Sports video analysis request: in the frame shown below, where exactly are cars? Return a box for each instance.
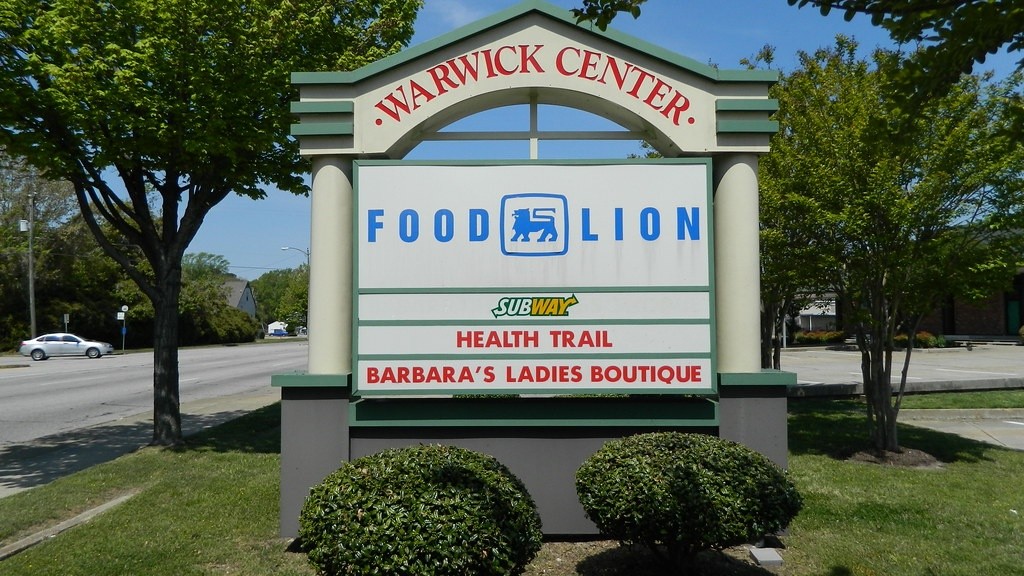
[19,333,114,361]
[270,329,288,337]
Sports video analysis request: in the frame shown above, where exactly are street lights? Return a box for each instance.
[281,246,310,286]
